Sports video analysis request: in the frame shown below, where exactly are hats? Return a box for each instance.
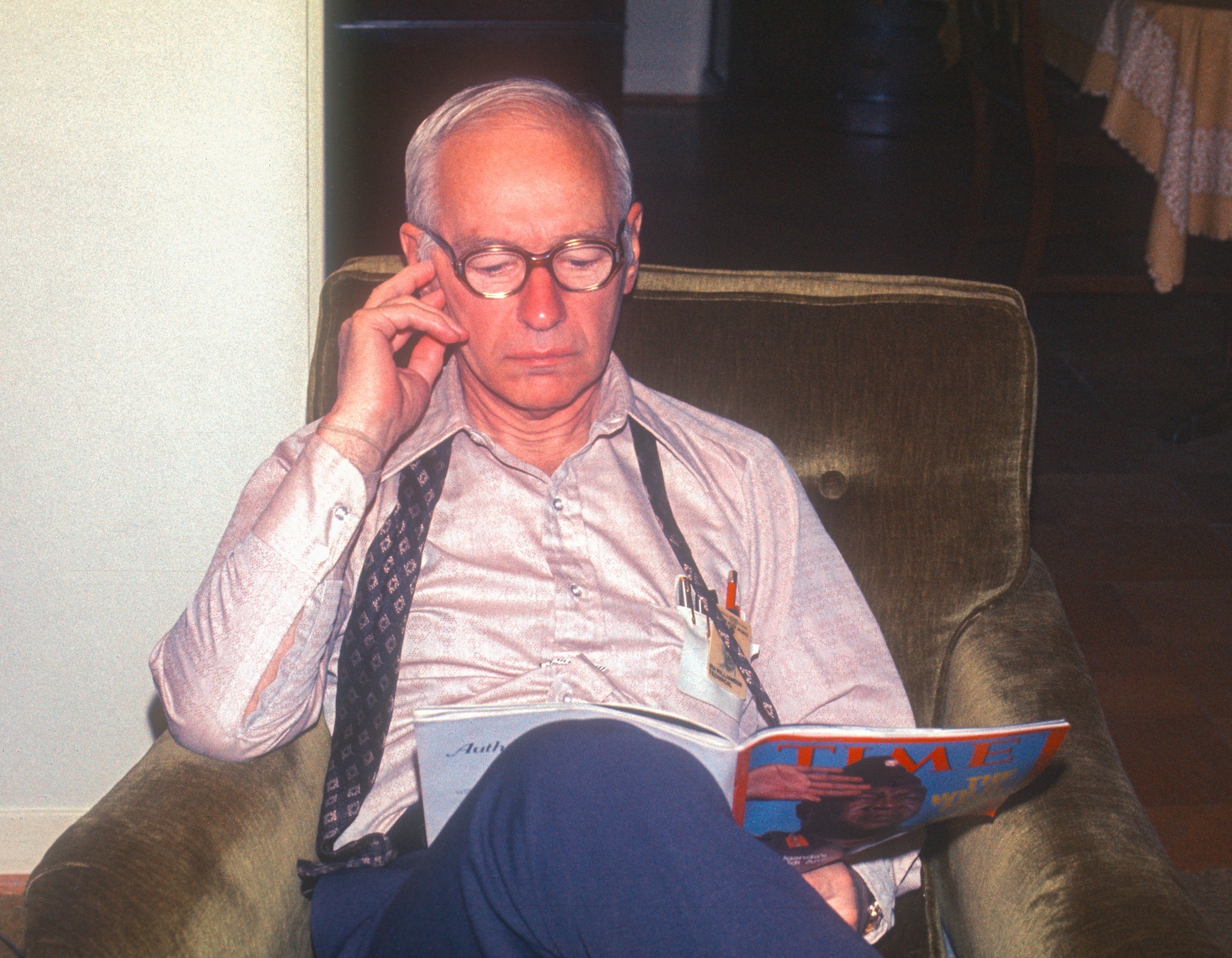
[837,754,921,786]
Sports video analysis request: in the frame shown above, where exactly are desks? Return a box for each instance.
[935,0,1232,294]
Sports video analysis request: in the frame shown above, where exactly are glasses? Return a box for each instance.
[417,221,628,302]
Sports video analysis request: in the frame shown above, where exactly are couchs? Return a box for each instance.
[14,253,1213,958]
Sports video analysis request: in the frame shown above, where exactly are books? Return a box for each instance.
[412,703,1070,874]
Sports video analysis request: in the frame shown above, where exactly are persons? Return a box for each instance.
[148,78,922,957]
[747,757,927,856]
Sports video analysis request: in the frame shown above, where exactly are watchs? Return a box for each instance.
[851,867,882,934]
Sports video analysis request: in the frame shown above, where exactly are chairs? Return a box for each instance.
[960,0,1144,311]
